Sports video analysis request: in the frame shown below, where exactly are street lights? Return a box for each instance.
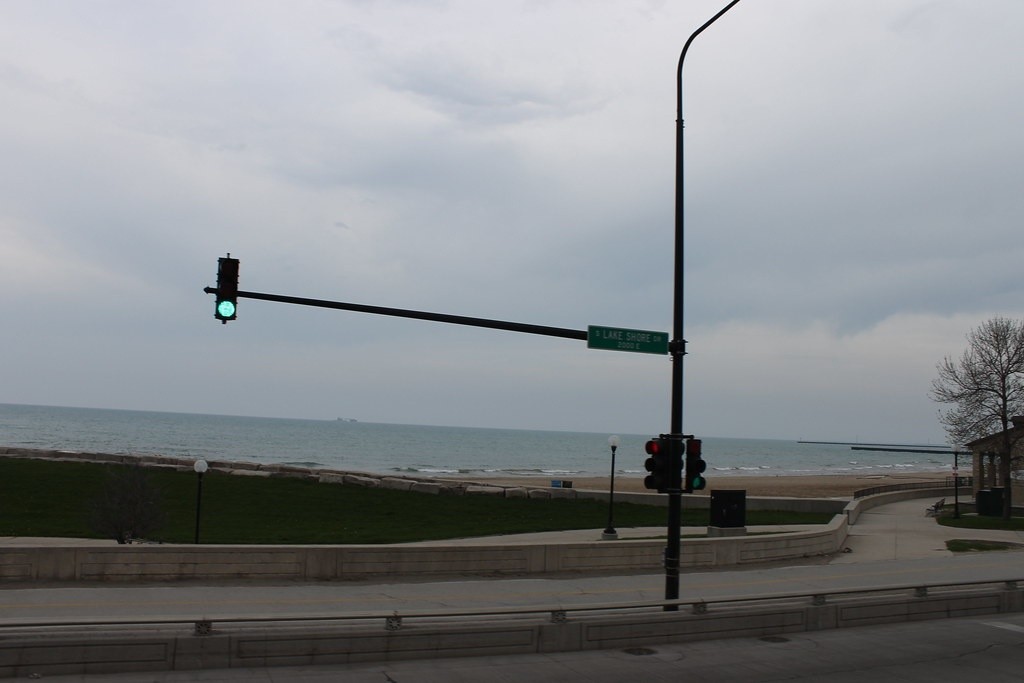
[603,434,619,534]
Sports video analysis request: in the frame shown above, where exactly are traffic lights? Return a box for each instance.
[684,437,708,494]
[644,437,670,495]
[214,256,240,322]
